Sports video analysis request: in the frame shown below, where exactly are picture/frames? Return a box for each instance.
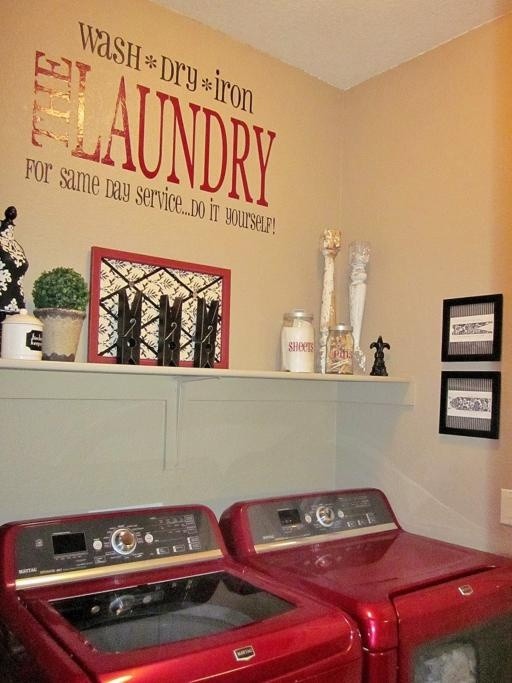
[438,370,501,440]
[440,292,504,361]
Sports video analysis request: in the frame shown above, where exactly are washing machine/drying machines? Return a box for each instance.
[216,486,512,683]
[0,505,364,683]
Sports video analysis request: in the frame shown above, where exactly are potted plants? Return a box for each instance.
[32,266,91,362]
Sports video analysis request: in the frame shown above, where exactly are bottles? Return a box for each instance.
[279,308,317,376]
[323,320,354,377]
[0,306,45,362]
